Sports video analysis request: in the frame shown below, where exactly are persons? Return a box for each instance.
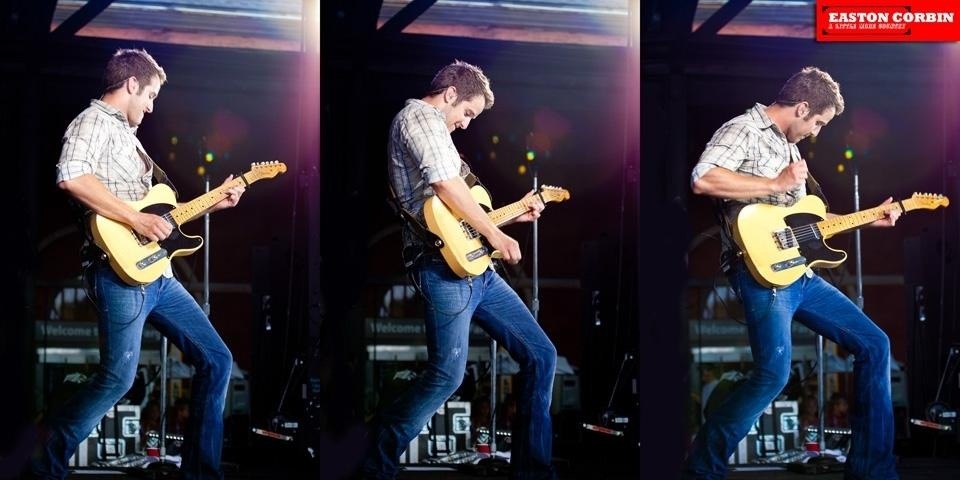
[366,60,561,479]
[680,67,906,480]
[32,46,248,480]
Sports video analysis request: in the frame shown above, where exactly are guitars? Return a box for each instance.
[732,191,950,290]
[424,184,570,278]
[90,160,287,286]
